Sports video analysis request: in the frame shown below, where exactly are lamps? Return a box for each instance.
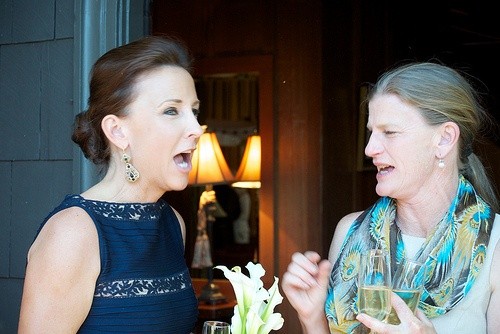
[232,131,261,269]
[186,131,236,305]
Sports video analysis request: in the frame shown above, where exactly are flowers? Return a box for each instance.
[215,264,286,332]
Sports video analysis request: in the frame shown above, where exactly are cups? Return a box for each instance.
[389,258,425,325]
[203,321,230,334]
[356,249,390,334]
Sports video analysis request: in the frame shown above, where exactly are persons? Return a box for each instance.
[18,36,203,334]
[282,62,500,334]
[167,185,258,278]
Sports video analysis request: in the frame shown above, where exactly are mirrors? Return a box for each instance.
[179,64,275,283]
